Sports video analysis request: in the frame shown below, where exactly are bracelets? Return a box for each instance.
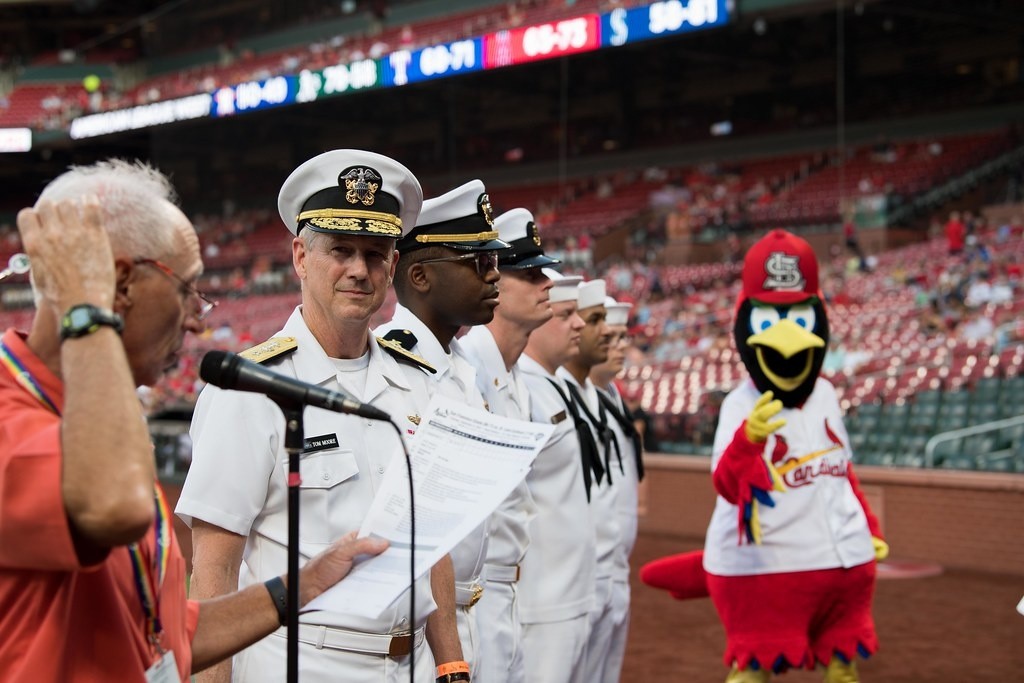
[435,661,470,678]
[436,672,470,683]
[265,577,289,627]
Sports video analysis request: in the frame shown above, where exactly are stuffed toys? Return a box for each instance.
[639,229,891,683]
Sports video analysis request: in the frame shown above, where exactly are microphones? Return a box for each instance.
[199,350,392,423]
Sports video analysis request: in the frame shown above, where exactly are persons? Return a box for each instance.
[607,264,743,360]
[648,161,773,238]
[0,148,645,683]
[910,209,1024,353]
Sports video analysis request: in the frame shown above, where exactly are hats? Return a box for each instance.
[603,296,633,326]
[278,149,424,244]
[575,279,607,310]
[541,267,583,303]
[489,207,562,272]
[397,180,513,251]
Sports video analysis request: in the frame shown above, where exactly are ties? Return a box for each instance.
[549,378,603,504]
[567,381,625,487]
[594,386,645,483]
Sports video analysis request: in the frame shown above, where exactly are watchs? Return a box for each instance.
[59,304,126,340]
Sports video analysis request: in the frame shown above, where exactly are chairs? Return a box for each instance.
[623,232,1024,472]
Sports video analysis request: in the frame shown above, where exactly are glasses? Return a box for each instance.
[129,257,219,321]
[415,249,498,279]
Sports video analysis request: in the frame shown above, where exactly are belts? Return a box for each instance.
[271,622,425,663]
[477,564,521,584]
[454,579,484,606]
